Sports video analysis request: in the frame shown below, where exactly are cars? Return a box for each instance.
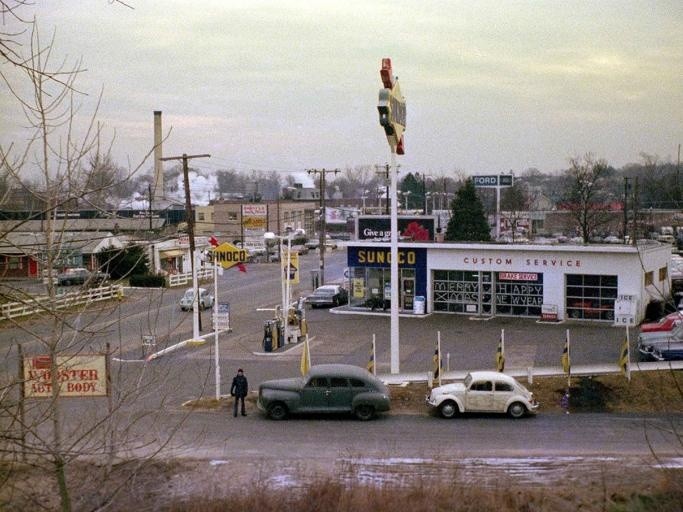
[304,284,348,309]
[424,371,541,419]
[490,218,683,245]
[41,267,111,287]
[236,238,341,263]
[255,363,391,421]
[636,309,683,361]
[179,287,215,312]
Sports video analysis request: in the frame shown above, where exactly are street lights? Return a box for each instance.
[263,228,306,346]
[361,190,447,216]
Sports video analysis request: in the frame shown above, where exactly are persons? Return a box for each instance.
[229,367,249,417]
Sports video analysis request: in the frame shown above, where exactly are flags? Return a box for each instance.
[560,336,571,377]
[429,331,447,385]
[494,331,506,371]
[617,324,632,379]
[299,337,311,375]
[367,340,375,374]
